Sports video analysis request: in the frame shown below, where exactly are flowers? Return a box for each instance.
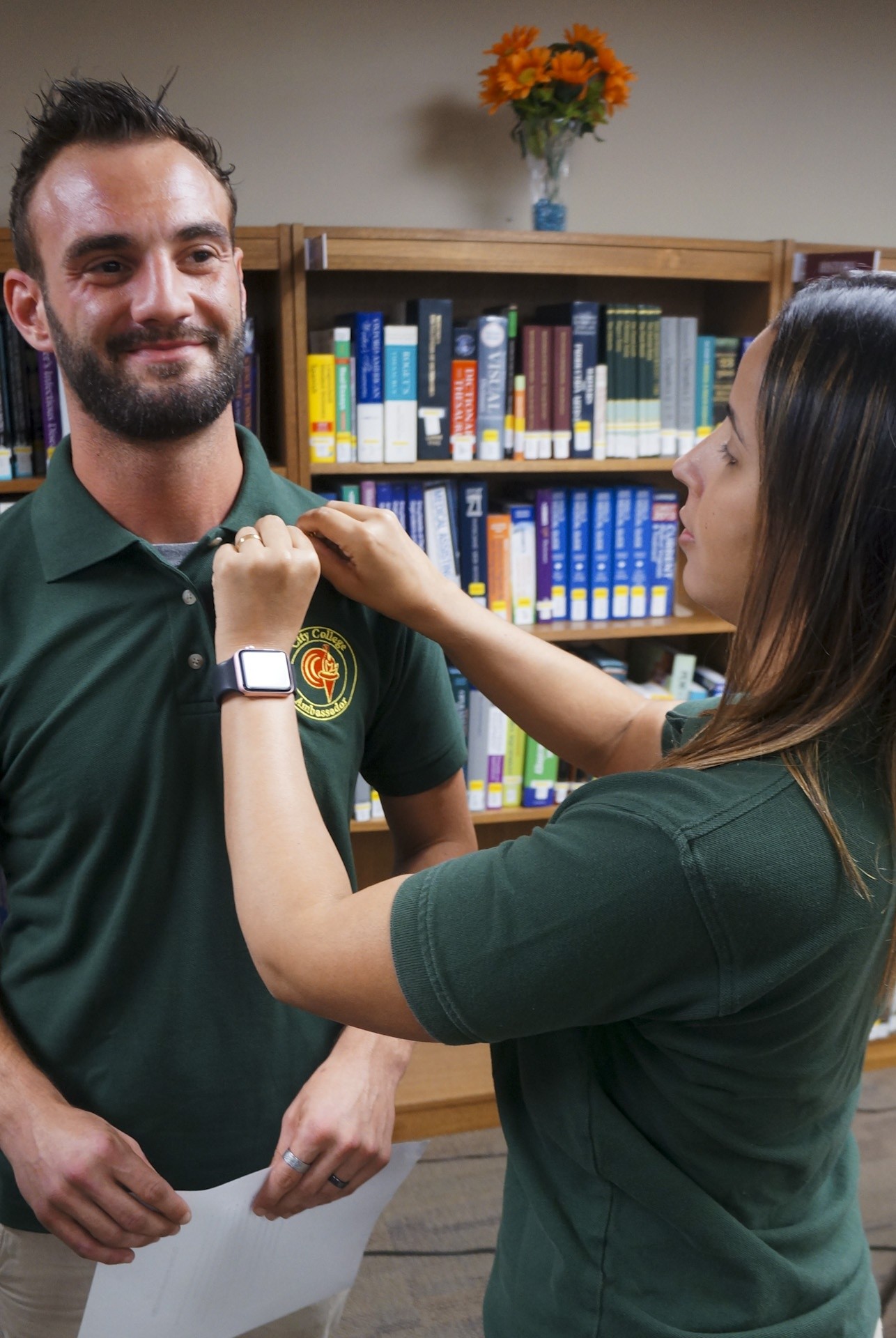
[476,21,640,204]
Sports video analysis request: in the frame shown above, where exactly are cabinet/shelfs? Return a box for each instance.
[0,223,896,1144]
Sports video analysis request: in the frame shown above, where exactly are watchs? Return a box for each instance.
[213,645,296,706]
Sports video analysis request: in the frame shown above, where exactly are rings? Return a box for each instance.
[235,534,262,552]
[282,1148,311,1175]
[328,1174,348,1189]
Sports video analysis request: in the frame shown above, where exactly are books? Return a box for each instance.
[303,473,696,624]
[0,272,282,483]
[306,274,766,465]
[346,644,731,824]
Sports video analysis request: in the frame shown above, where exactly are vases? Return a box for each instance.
[519,119,581,231]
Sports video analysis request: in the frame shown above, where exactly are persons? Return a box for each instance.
[0,75,479,1338]
[211,266,896,1338]
